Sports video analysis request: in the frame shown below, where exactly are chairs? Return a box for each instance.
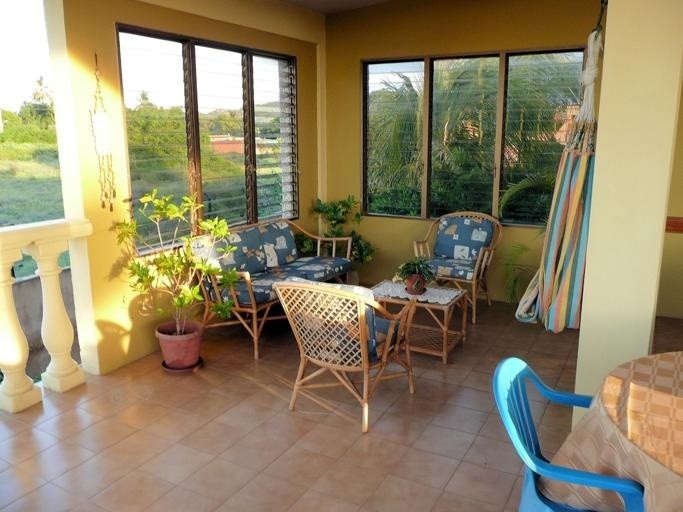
[493,357,645,512]
[413,211,503,324]
[272,276,417,432]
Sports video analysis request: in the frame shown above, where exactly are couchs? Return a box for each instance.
[183,218,353,360]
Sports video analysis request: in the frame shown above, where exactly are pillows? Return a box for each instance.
[433,216,494,262]
[283,276,376,356]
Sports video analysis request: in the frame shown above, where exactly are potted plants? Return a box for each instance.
[392,255,434,294]
[112,189,239,375]
